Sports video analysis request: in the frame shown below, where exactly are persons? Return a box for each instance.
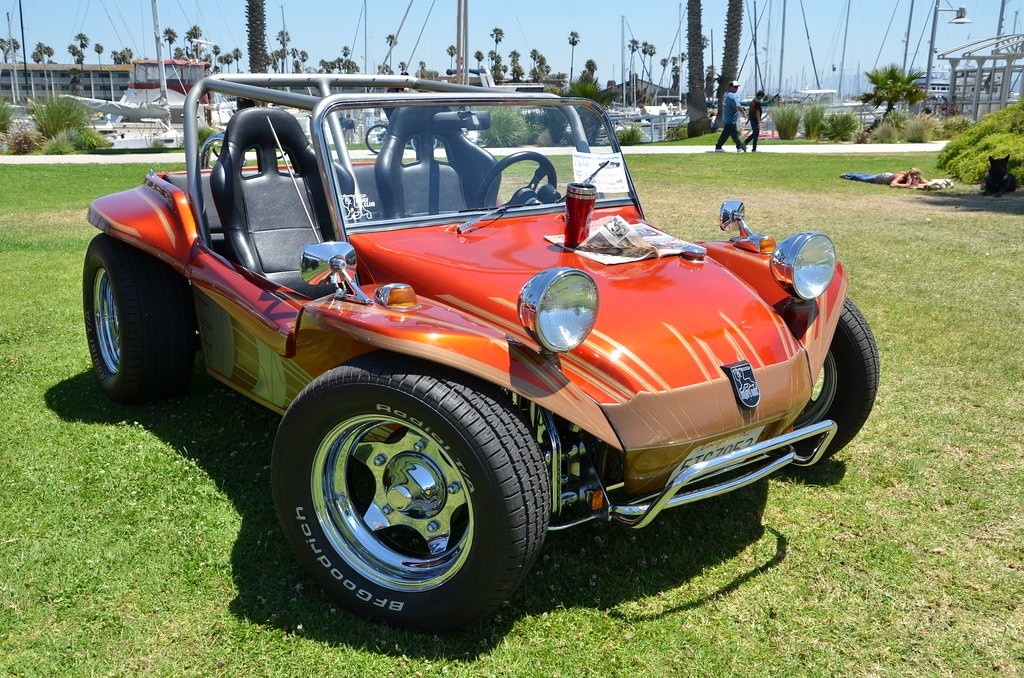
[714,80,748,152]
[839,167,928,188]
[742,90,765,152]
[338,112,355,144]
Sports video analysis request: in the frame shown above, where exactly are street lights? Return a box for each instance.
[924,6,973,108]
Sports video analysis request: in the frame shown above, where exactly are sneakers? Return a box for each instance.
[715,148,725,152]
[737,148,744,154]
[743,144,748,152]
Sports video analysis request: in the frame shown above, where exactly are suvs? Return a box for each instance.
[83,72,881,635]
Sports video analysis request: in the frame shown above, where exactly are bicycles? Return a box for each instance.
[365,124,437,155]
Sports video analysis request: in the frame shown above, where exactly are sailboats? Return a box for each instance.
[1,0,1024,149]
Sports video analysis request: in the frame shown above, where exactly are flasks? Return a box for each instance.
[565,183,596,248]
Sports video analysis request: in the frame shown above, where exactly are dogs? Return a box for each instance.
[981,153,1016,197]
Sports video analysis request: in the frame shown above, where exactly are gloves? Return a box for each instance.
[742,113,746,120]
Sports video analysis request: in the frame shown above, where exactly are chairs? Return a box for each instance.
[372,106,503,220]
[209,107,355,300]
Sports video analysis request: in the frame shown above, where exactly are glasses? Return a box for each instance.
[731,86,738,88]
[912,175,921,180]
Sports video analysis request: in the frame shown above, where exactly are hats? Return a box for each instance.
[758,90,765,94]
[729,81,740,87]
